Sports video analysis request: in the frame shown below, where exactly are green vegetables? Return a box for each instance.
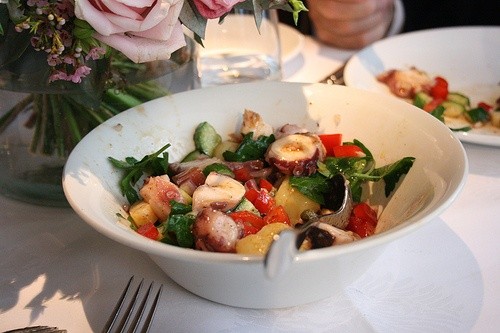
[107,132,416,250]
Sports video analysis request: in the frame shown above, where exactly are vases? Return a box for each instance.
[0,56,192,207]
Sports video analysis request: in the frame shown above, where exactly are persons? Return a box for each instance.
[274,0,500,49]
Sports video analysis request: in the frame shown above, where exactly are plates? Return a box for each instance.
[181,14,305,77]
[343,25,500,149]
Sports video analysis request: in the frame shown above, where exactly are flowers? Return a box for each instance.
[0,0,310,97]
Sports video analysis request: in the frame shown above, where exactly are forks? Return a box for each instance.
[102,274,165,333]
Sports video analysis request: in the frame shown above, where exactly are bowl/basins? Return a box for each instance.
[58,82,468,309]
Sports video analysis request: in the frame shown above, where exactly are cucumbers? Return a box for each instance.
[415,90,490,133]
[193,121,222,157]
